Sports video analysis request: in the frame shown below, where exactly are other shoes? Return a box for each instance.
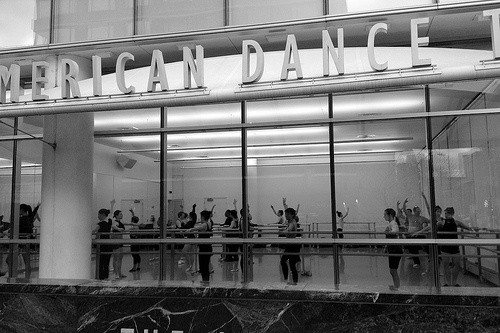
[442,283,461,286]
[248,260,254,265]
[230,268,239,272]
[115,272,127,279]
[2,272,9,278]
[218,257,239,262]
[129,267,140,272]
[413,264,418,267]
[178,259,184,265]
[186,265,192,272]
[210,270,214,274]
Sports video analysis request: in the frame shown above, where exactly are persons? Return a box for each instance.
[0,196,479,291]
